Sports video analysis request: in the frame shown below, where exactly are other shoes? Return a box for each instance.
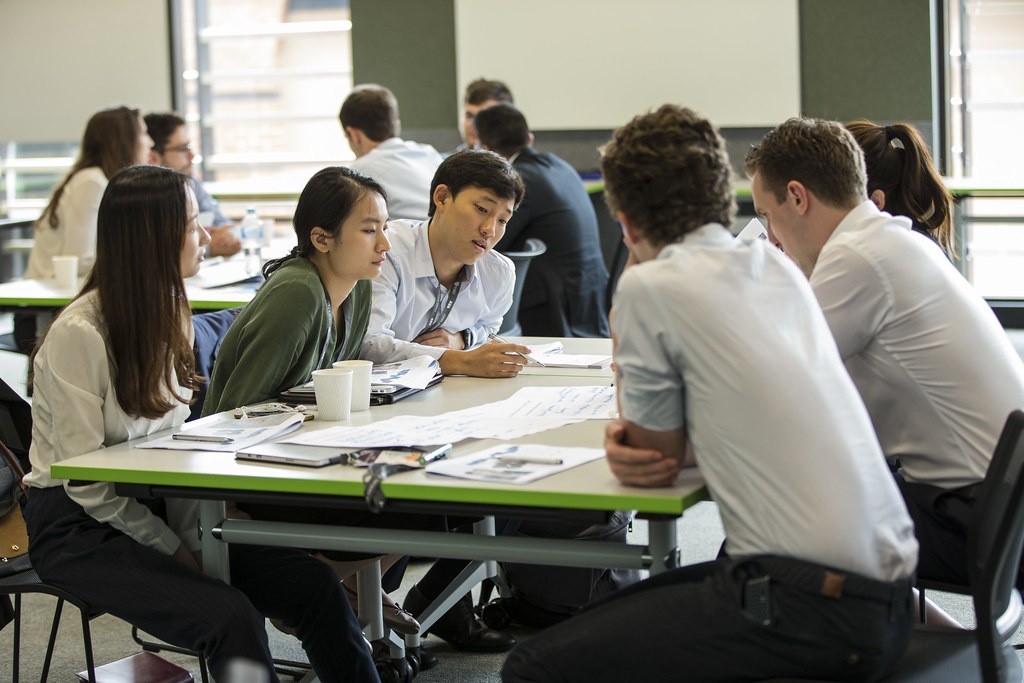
[341,587,420,633]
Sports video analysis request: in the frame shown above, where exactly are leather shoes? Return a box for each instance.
[400,585,517,651]
[395,629,439,670]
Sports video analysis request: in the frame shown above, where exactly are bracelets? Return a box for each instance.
[459,328,472,351]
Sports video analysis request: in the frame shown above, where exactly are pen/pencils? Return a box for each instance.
[417,444,453,465]
[489,333,545,366]
[496,455,563,463]
[172,435,235,442]
[544,362,603,370]
[611,374,617,387]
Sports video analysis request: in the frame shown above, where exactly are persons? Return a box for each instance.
[336,154,518,651]
[465,102,614,342]
[741,113,1023,643]
[138,113,243,260]
[494,99,918,683]
[438,81,524,182]
[184,155,424,653]
[328,85,446,226]
[20,162,392,681]
[10,106,218,361]
[839,120,950,261]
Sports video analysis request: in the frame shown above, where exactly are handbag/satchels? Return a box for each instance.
[0,437,36,576]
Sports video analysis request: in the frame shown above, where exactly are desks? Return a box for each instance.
[0,239,303,334]
[732,177,1024,283]
[50,337,709,683]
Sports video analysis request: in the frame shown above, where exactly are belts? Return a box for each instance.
[764,556,917,604]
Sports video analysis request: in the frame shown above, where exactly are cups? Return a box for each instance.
[311,369,354,422]
[332,360,373,412]
[53,256,80,295]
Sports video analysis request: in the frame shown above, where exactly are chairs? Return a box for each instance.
[497,238,547,337]
[880,410,1024,683]
[0,379,207,683]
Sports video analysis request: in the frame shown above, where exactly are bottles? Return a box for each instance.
[240,209,264,275]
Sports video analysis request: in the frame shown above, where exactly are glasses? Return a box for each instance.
[159,141,194,154]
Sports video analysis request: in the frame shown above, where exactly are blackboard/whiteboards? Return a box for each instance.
[454,0,803,131]
